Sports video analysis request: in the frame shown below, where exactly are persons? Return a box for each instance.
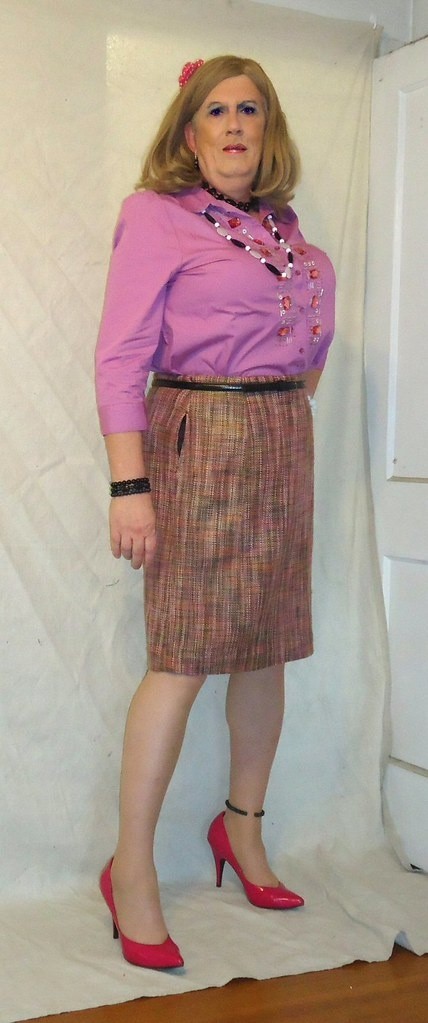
[94,54,335,968]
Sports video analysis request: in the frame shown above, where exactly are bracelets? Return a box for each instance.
[111,477,151,496]
[307,395,318,416]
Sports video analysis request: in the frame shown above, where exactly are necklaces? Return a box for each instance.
[203,209,294,279]
[200,182,257,214]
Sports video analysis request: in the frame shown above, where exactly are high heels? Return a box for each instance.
[98,855,185,967]
[208,812,304,908]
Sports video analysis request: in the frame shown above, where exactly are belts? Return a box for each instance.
[151,378,305,392]
